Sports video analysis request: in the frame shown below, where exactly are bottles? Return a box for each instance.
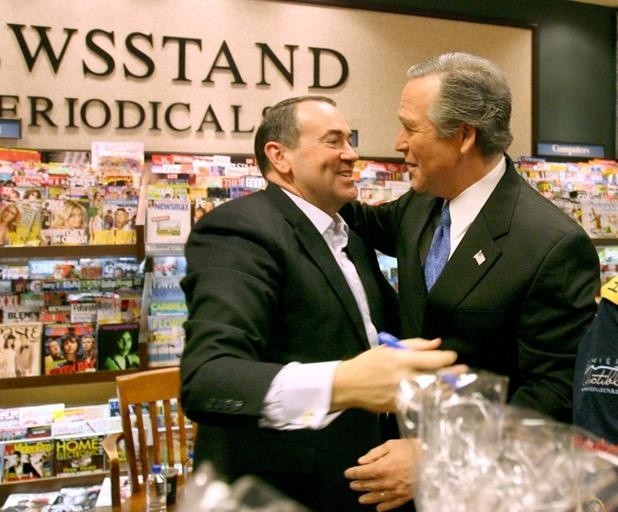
[146,465,167,512]
[184,449,194,496]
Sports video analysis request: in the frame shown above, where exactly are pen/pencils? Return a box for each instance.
[377,331,463,390]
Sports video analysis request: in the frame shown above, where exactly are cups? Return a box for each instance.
[160,469,178,505]
[109,398,120,417]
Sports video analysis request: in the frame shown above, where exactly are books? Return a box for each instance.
[0,394,197,511]
[2,254,190,381]
[352,157,618,292]
[0,140,266,245]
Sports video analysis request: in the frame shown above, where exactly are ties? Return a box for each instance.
[424,200,450,294]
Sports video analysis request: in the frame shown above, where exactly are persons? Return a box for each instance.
[573,274,618,455]
[179,95,469,512]
[339,51,601,512]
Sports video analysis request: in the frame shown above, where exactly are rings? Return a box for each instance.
[380,492,386,501]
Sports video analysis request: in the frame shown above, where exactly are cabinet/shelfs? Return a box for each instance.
[1,145,618,512]
